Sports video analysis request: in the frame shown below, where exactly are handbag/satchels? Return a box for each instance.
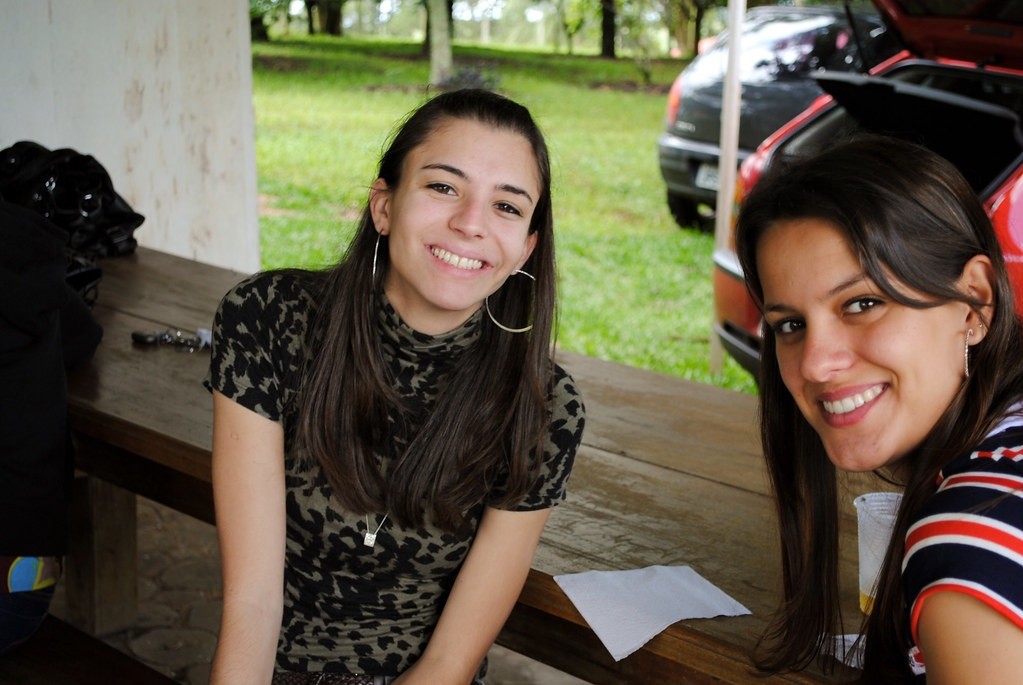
[0,140,144,258]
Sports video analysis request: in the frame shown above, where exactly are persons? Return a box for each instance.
[734,135,1023,685]
[203,90,586,685]
[0,200,102,651]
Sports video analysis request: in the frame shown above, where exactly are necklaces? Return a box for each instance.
[364,512,388,547]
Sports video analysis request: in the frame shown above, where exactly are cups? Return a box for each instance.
[854,494,910,613]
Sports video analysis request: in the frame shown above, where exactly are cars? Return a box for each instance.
[712,1,1023,391]
[656,4,886,231]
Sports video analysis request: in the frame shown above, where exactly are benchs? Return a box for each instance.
[0,245,926,685]
